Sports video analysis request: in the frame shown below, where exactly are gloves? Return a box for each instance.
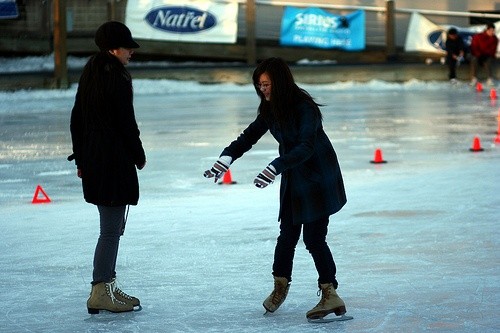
[253,165,278,189]
[133,144,146,170]
[203,158,231,183]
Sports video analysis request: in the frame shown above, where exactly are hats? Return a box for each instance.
[94,21,140,52]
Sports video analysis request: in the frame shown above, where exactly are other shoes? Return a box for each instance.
[470,76,477,86]
[487,77,495,87]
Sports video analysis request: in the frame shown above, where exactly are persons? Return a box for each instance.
[469,22,498,86]
[445,28,466,84]
[204,57,347,319]
[70,21,146,314]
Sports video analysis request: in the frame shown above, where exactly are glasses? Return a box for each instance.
[257,82,271,89]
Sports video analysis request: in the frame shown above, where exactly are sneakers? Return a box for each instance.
[262,276,291,317]
[306,283,354,323]
[86,282,143,318]
